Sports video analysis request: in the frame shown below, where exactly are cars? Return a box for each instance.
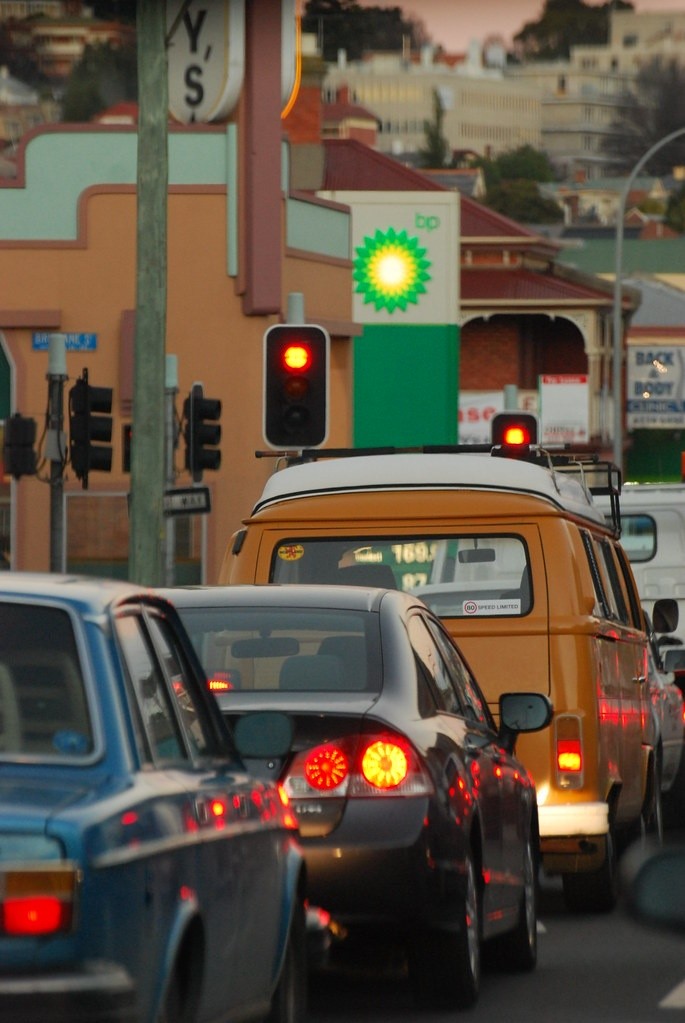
[403,580,685,794]
[0,567,313,1022]
[153,584,558,1010]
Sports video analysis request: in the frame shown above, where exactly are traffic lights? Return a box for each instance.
[262,324,330,450]
[119,422,132,473]
[182,382,223,485]
[489,409,541,464]
[0,411,38,481]
[67,366,115,492]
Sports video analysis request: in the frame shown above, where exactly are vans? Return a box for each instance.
[427,479,684,628]
[222,435,680,915]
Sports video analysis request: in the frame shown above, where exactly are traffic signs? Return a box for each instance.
[127,487,213,518]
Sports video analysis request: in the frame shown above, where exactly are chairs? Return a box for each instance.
[12,665,72,724]
[208,669,240,691]
[308,565,399,591]
[279,636,370,690]
